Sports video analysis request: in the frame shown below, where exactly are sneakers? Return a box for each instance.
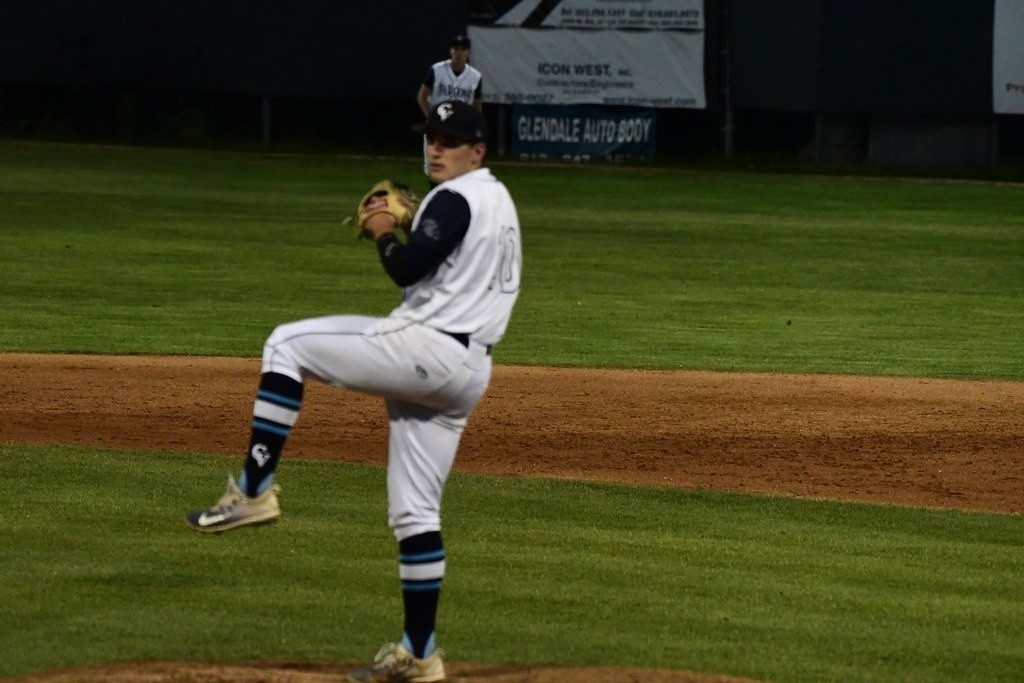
[184,476,279,535]
[346,642,445,683]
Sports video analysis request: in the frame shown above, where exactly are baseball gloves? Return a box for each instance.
[357,179,420,232]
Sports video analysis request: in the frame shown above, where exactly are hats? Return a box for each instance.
[414,100,485,143]
[448,35,471,47]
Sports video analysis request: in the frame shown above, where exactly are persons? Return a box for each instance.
[416,33,487,195]
[181,98,527,683]
